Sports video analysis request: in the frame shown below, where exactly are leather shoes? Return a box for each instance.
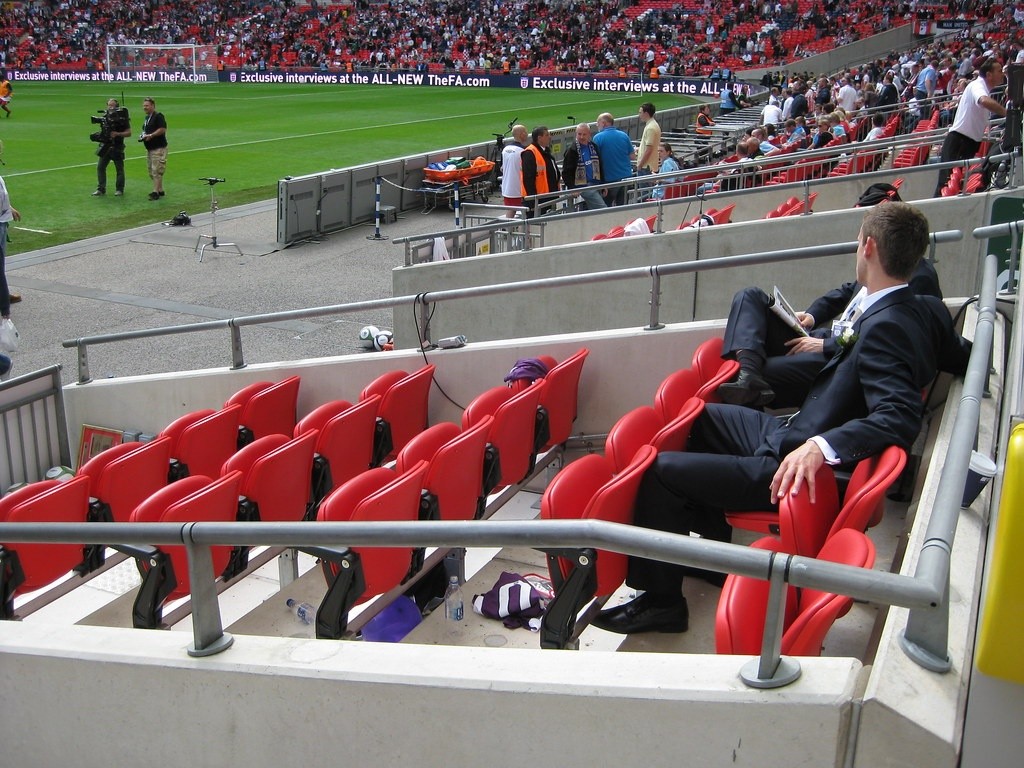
[718,370,777,406]
[589,592,689,634]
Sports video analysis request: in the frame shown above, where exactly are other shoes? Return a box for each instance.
[148,191,164,201]
[9,292,22,304]
[115,191,123,195]
[93,190,102,195]
[6,111,11,117]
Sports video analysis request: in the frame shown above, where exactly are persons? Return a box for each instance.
[0,353,13,381]
[500,124,528,240]
[590,202,974,634]
[592,112,636,207]
[520,126,560,219]
[0,0,1024,88]
[562,123,608,210]
[720,82,739,115]
[694,104,715,157]
[0,75,12,119]
[139,97,168,201]
[696,0,1024,193]
[0,245,13,321]
[933,57,1006,197]
[637,102,661,202]
[737,84,752,106]
[712,255,944,403]
[0,175,22,303]
[91,98,133,197]
[651,142,678,200]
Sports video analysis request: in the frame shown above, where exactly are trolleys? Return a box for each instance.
[420,156,496,215]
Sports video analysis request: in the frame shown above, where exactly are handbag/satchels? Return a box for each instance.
[0,317,20,352]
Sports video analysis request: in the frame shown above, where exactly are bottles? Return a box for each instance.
[286,598,317,625]
[443,576,464,638]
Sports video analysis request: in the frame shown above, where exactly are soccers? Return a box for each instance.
[359,326,379,349]
[374,330,393,352]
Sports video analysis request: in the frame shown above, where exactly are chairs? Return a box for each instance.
[604,396,704,476]
[223,375,300,452]
[0,475,90,620]
[157,403,242,482]
[219,427,318,522]
[779,443,909,558]
[726,511,780,535]
[108,469,243,631]
[691,338,725,385]
[461,377,546,495]
[395,414,493,521]
[716,528,876,657]
[292,393,382,510]
[0,0,1024,244]
[359,362,436,467]
[541,444,657,600]
[77,434,173,522]
[508,346,590,453]
[653,359,740,426]
[294,460,428,639]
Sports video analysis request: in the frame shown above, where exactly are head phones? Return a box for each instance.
[114,99,119,107]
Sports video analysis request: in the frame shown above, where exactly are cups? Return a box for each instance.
[959,450,998,510]
